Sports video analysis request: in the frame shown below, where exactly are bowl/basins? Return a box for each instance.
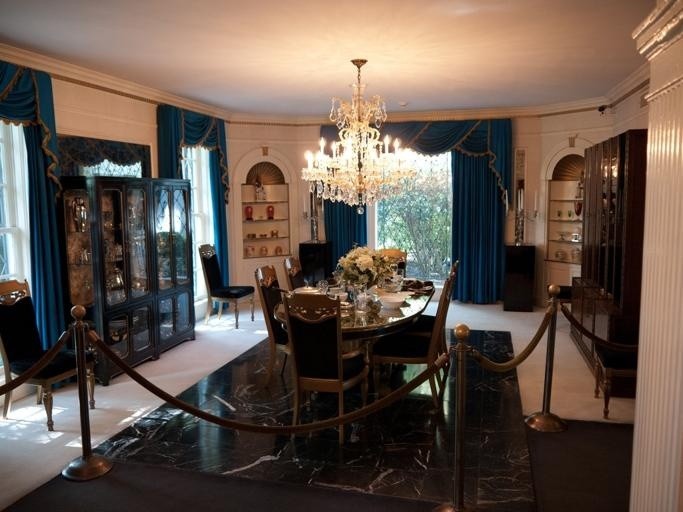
[380,295,406,309]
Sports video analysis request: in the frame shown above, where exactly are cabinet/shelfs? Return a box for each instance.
[580,126,648,314]
[545,179,584,266]
[241,181,291,260]
[150,176,197,360]
[54,172,155,385]
[566,276,640,399]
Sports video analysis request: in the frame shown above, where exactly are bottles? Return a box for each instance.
[555,208,572,218]
[247,245,283,256]
[248,229,280,237]
[254,181,268,201]
[244,206,276,220]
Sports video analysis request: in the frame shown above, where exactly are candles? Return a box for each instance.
[301,188,325,216]
[503,186,538,211]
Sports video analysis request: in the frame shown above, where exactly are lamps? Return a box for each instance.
[299,58,423,215]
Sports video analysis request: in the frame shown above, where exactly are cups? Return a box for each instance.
[317,264,397,308]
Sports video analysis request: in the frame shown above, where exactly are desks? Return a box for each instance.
[502,245,537,313]
[297,239,333,284]
[273,275,435,410]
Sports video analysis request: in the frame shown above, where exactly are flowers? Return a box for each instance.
[336,248,391,302]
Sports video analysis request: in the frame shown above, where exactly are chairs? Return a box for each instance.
[283,258,304,289]
[198,243,255,330]
[371,277,455,419]
[403,259,458,376]
[256,265,291,385]
[379,248,408,278]
[282,293,371,452]
[0,278,97,434]
[591,340,641,419]
